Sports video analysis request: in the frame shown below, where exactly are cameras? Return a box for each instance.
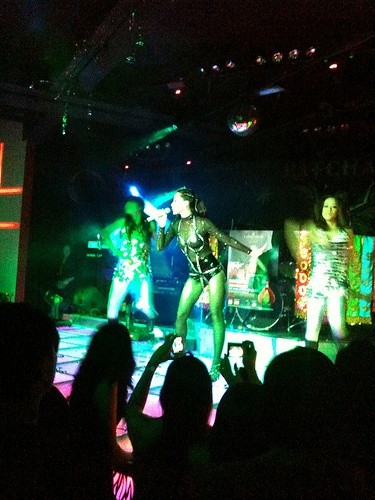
[227,342,252,358]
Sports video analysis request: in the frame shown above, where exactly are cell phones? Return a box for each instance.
[171,336,186,359]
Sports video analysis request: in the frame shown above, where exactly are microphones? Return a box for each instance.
[144,207,171,224]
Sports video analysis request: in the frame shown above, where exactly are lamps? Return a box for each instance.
[225,92,259,137]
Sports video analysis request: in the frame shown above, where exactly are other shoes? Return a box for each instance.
[210,357,220,381]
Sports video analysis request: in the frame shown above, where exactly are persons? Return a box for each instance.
[0,301,375,500]
[103,196,160,341]
[285,192,358,351]
[155,189,268,382]
[23,242,113,318]
